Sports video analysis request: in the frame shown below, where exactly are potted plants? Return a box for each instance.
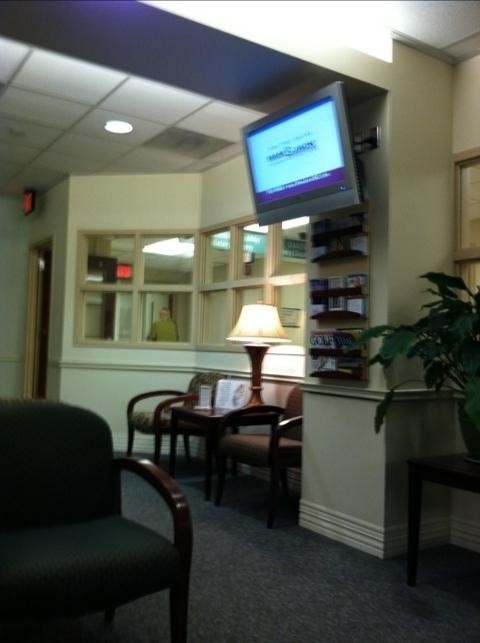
[344,268,480,458]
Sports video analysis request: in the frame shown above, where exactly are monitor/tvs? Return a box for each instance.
[238,80,361,228]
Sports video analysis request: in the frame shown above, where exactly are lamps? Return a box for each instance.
[224,304,293,409]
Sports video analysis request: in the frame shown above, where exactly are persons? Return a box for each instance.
[146,308,178,343]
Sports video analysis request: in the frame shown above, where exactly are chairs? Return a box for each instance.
[213,385,305,529]
[0,398,192,642]
[126,371,226,463]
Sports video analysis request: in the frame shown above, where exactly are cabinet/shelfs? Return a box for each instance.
[305,209,374,380]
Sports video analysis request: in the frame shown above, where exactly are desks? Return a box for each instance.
[167,406,277,502]
[403,450,480,587]
